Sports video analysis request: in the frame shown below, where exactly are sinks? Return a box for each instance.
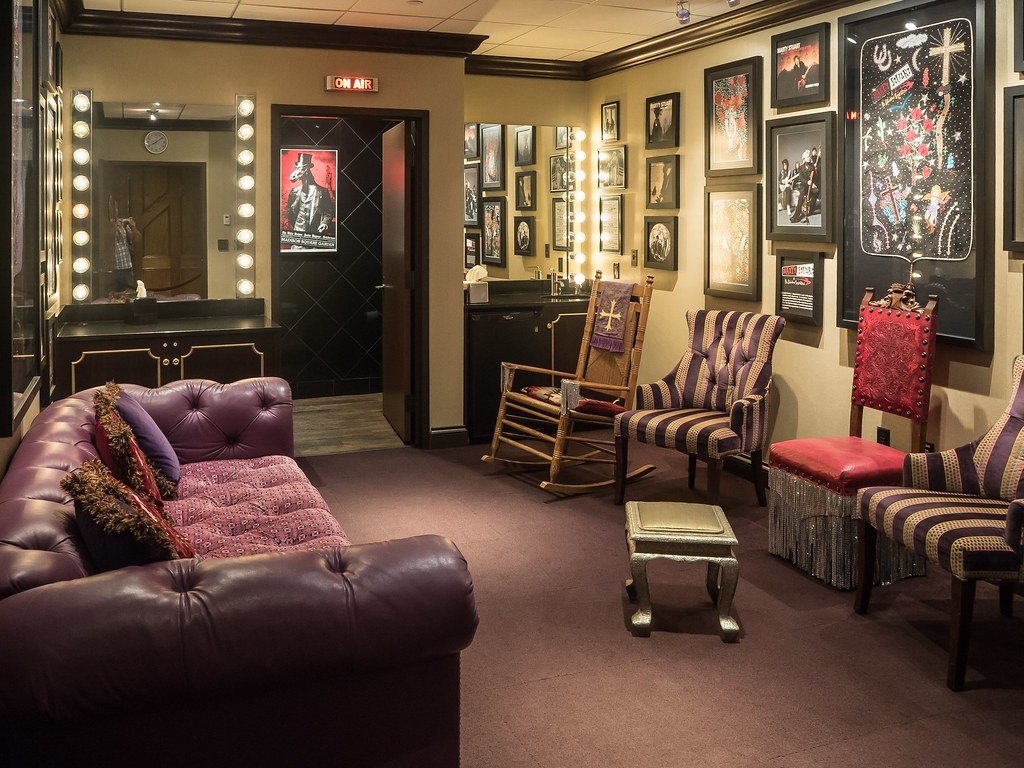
[541,294,591,300]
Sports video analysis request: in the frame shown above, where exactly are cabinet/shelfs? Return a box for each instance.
[465,309,621,442]
[63,332,280,402]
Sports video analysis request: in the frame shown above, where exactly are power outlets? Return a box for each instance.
[877,426,890,447]
[925,442,935,453]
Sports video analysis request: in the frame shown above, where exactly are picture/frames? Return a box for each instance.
[0,0,64,438]
[645,0,1024,371]
[464,124,567,268]
[602,102,619,142]
[597,145,627,189]
[599,195,623,256]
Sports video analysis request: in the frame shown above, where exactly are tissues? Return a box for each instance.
[465,264,489,302]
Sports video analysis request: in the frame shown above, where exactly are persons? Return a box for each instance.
[286,153,334,250]
[777,147,821,223]
[114,200,141,292]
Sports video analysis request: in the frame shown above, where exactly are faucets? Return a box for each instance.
[527,265,543,280]
[551,272,565,295]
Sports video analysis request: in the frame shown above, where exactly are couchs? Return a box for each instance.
[0,375,475,768]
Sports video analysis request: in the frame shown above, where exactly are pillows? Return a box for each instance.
[64,381,193,562]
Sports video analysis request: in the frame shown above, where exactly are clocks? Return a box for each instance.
[144,132,168,154]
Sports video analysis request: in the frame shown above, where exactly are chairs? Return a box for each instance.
[479,270,1024,690]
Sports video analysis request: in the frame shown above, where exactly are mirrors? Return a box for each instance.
[88,104,235,305]
[463,123,574,280]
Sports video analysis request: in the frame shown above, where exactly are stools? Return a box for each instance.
[624,501,742,643]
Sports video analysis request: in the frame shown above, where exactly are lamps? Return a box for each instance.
[846,31,861,45]
[676,0,690,24]
[905,18,919,29]
